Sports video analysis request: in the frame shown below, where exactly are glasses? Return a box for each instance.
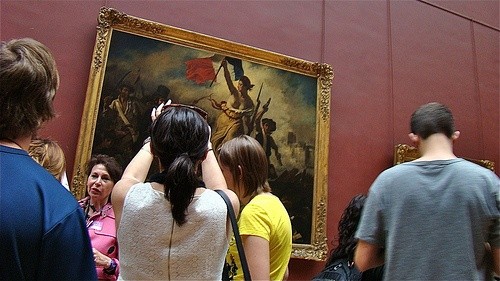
[161,104,208,121]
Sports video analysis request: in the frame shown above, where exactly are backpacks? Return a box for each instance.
[311,257,362,281]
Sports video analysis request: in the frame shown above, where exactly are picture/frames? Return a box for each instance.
[393,143,497,175]
[70,4,336,265]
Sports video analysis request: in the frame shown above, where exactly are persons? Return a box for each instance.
[77,154,120,281]
[354,102,500,281]
[326,194,384,281]
[29,139,65,182]
[0,37,99,281]
[111,100,240,281]
[218,136,293,281]
